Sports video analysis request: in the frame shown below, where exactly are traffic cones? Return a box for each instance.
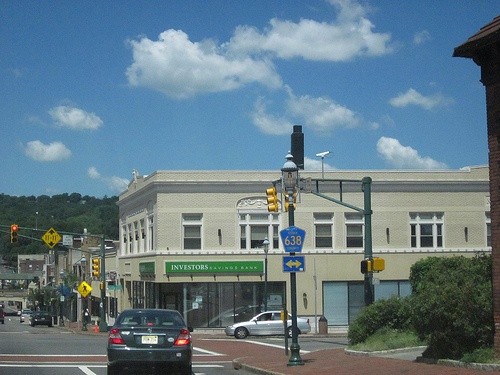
[94,325,99,333]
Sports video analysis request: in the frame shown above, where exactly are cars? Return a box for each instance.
[0,304,21,324]
[21,309,52,327]
[107,308,194,375]
[224,311,311,338]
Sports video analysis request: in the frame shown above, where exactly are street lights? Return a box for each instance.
[280,151,304,366]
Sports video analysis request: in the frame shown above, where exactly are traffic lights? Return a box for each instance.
[361,260,371,273]
[10,224,19,244]
[265,186,278,212]
[100,283,103,289]
[285,192,297,211]
[92,257,100,278]
